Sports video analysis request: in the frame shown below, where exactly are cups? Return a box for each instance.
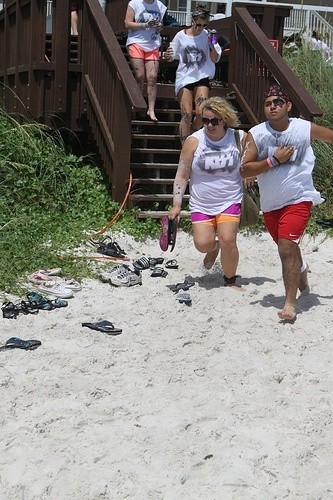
[170,42,179,59]
[210,29,218,44]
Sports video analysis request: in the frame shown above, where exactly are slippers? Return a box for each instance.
[150,267,168,278]
[166,284,180,291]
[177,294,191,303]
[178,281,193,290]
[82,320,122,335]
[133,256,152,269]
[160,216,177,252]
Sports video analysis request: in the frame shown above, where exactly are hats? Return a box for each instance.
[262,84,288,102]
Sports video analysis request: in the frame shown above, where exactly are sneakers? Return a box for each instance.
[109,270,142,287]
[38,279,81,298]
[100,264,130,283]
[87,237,100,249]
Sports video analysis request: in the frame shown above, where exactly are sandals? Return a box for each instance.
[16,301,39,315]
[27,292,53,310]
[49,299,68,307]
[5,338,41,348]
[98,237,127,258]
[148,257,164,265]
[2,302,18,318]
[166,259,178,269]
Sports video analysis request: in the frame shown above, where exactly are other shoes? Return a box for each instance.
[27,269,62,283]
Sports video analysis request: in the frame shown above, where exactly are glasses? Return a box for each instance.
[194,19,208,28]
[201,117,223,126]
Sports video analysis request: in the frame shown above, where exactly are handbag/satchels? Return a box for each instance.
[238,188,260,230]
